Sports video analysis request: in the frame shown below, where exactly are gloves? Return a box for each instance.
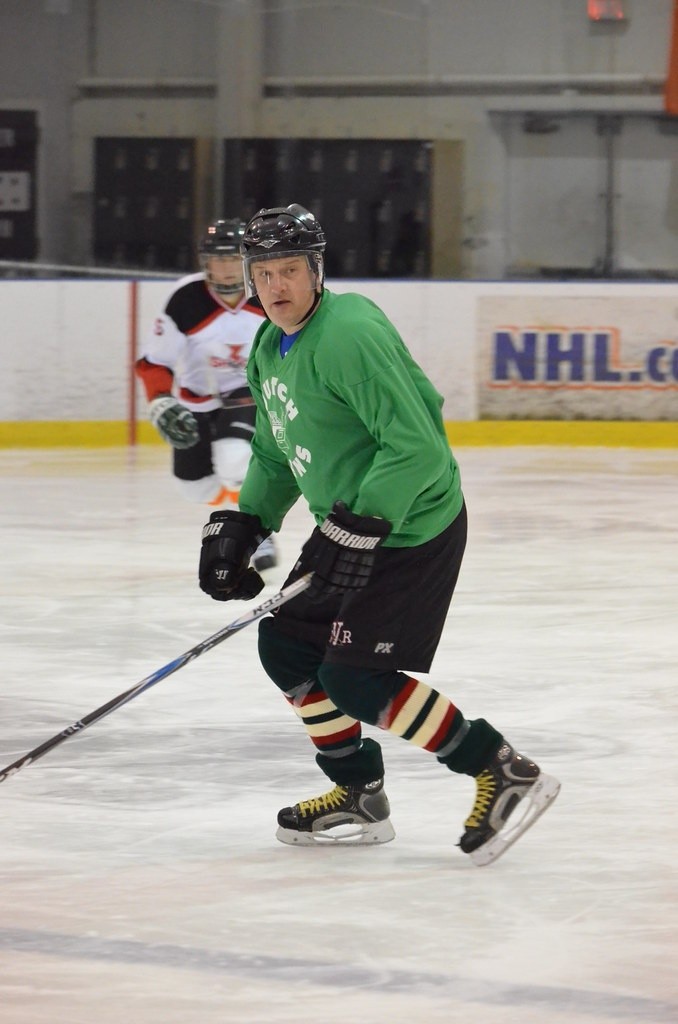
[147,395,200,450]
[197,508,272,603]
[292,500,393,606]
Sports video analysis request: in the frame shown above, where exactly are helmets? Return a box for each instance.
[239,203,327,262]
[195,218,247,257]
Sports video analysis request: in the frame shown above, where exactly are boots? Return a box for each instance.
[275,738,396,848]
[436,717,563,871]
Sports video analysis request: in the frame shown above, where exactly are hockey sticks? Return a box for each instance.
[0,568,314,783]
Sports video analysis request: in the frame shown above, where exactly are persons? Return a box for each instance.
[135,217,278,584]
[198,202,561,870]
